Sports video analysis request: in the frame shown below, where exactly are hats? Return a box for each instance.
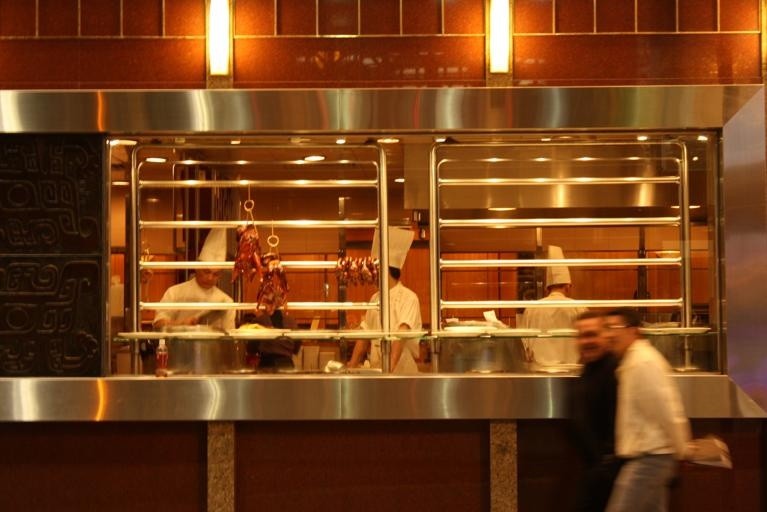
[197,228,227,263]
[544,245,573,291]
[370,225,416,271]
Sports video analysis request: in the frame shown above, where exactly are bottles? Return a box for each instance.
[156,338,170,377]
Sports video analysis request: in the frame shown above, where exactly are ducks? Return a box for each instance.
[230,223,263,286]
[255,252,290,316]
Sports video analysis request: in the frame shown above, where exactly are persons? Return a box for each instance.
[347,266,422,373]
[519,284,589,364]
[152,269,302,368]
[569,309,698,512]
